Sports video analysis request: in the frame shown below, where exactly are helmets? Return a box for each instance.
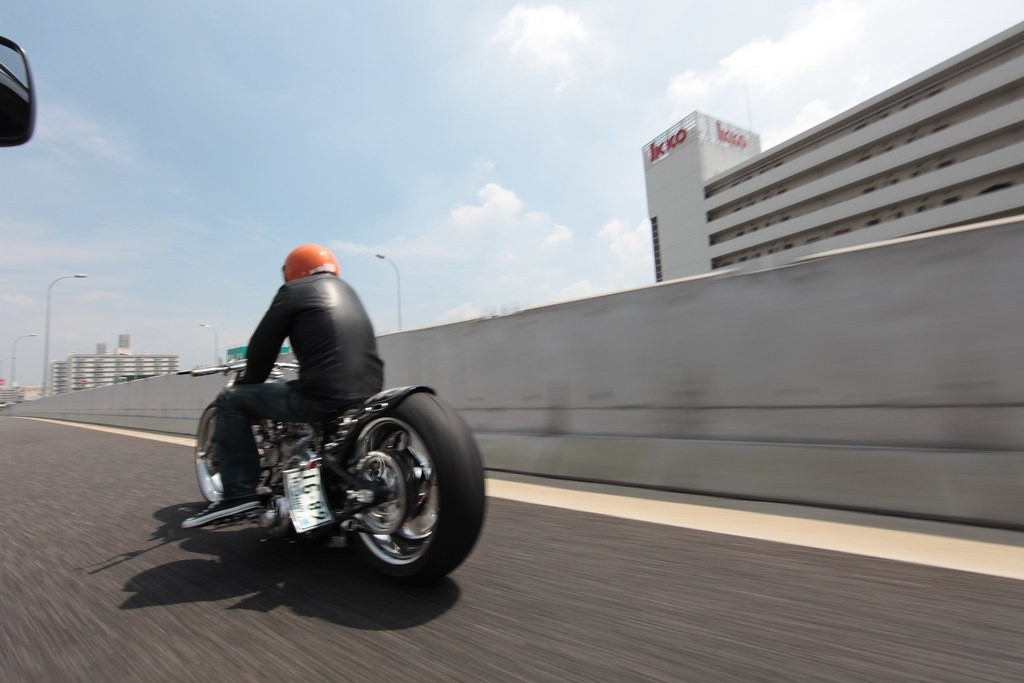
[281,243,340,281]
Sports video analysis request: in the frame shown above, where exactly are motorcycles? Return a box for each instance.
[175,354,489,586]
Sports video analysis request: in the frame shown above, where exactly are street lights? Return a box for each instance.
[376,252,406,337]
[42,273,87,396]
[10,333,38,383]
[198,322,220,366]
[0,356,17,384]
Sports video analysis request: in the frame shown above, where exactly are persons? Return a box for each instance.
[182,244,385,528]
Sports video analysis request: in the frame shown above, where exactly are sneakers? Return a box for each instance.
[181,491,264,529]
